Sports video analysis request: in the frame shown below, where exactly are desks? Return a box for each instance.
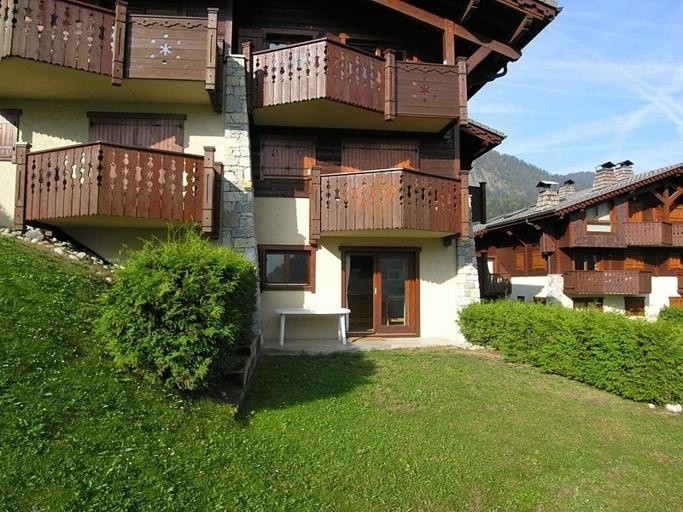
[383,298,405,326]
[275,308,352,346]
